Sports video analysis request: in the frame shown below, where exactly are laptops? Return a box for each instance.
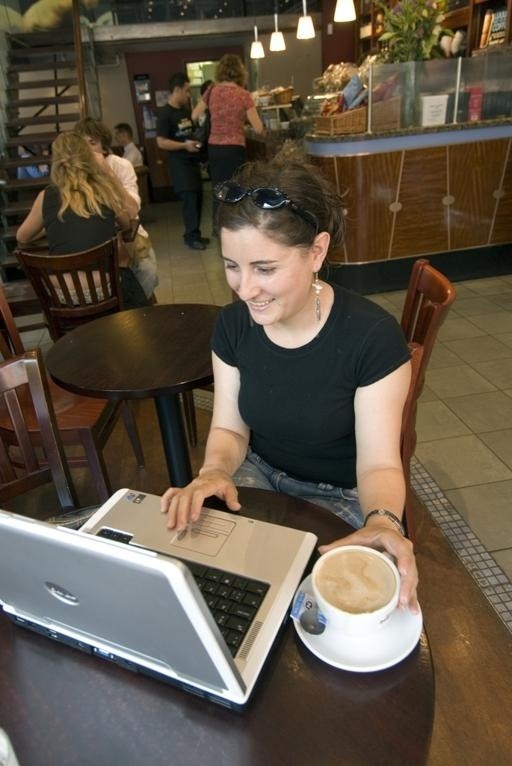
[0,487,319,718]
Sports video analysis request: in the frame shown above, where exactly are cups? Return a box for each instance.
[280,122,289,130]
[311,546,400,627]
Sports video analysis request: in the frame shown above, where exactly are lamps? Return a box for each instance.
[249,0,356,59]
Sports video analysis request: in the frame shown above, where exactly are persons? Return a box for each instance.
[156,73,210,249]
[73,115,160,298]
[158,158,418,613]
[17,142,47,180]
[190,54,266,238]
[113,123,144,169]
[16,131,149,307]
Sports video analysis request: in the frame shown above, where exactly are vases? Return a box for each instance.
[400,62,417,127]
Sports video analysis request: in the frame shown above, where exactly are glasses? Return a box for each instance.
[212,181,319,232]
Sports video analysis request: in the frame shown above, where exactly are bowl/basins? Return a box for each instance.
[259,96,272,106]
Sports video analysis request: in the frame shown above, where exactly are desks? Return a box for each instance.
[0,486,436,766]
[45,303,222,488]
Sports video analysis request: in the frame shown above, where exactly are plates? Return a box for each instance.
[292,573,424,672]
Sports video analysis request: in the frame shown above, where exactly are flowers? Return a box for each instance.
[375,0,456,79]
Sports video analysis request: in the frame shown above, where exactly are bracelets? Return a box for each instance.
[363,509,406,537]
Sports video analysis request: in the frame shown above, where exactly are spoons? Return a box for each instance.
[300,603,326,636]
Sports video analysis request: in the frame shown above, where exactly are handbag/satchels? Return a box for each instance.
[192,108,210,142]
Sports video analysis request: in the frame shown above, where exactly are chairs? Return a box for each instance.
[12,236,124,343]
[400,342,424,545]
[1,284,146,502]
[134,165,149,204]
[0,349,80,514]
[400,259,455,457]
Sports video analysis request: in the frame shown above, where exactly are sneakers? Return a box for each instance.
[200,238,209,244]
[188,240,205,250]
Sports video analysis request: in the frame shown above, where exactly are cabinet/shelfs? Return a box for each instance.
[130,74,173,188]
[355,0,511,64]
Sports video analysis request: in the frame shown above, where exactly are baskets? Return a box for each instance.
[371,97,400,130]
[315,107,366,136]
[275,88,294,104]
